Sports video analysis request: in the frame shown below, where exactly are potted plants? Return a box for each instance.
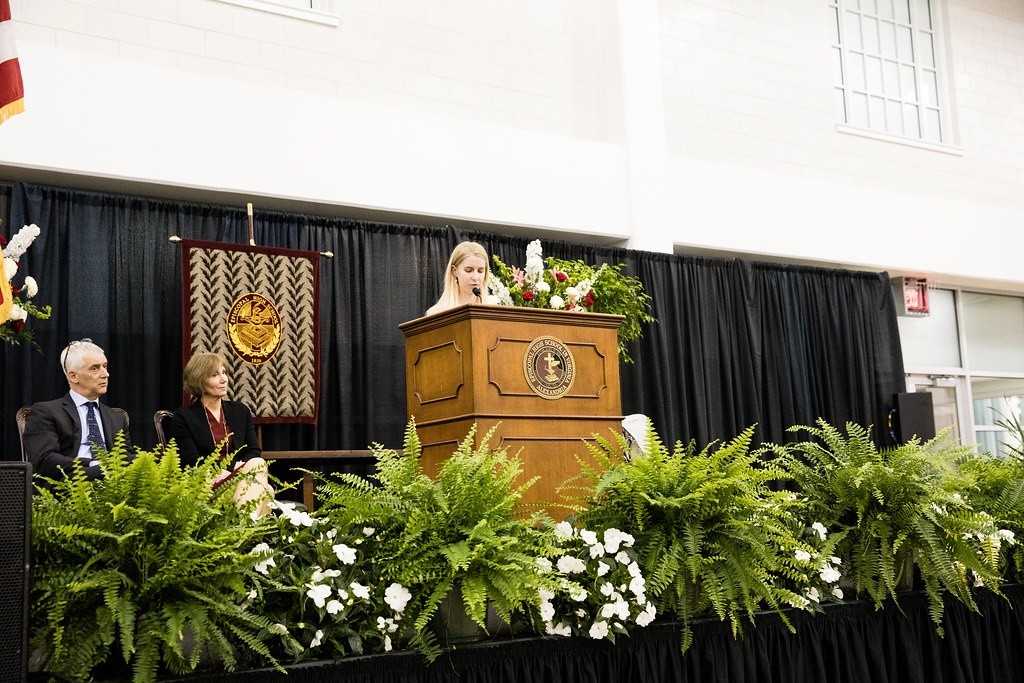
[760,391,1024,640]
[31,430,289,683]
[553,418,817,654]
[289,414,569,668]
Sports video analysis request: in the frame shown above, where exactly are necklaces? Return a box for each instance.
[202,399,229,456]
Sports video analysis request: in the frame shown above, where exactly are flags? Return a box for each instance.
[179,238,321,426]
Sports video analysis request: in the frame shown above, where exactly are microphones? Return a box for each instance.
[472,287,482,303]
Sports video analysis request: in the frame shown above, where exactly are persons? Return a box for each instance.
[422,241,514,318]
[171,353,276,523]
[20,341,149,605]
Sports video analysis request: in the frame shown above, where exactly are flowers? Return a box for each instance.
[741,494,844,609]
[0,216,53,354]
[245,498,412,663]
[492,238,659,367]
[929,495,1015,589]
[517,512,658,646]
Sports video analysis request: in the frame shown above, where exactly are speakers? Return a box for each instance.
[893,392,936,447]
[0,461,33,683]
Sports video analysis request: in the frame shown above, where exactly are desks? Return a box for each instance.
[259,450,403,513]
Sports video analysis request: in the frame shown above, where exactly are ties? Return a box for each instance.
[82,402,106,460]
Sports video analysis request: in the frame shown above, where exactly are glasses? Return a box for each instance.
[65,338,93,373]
